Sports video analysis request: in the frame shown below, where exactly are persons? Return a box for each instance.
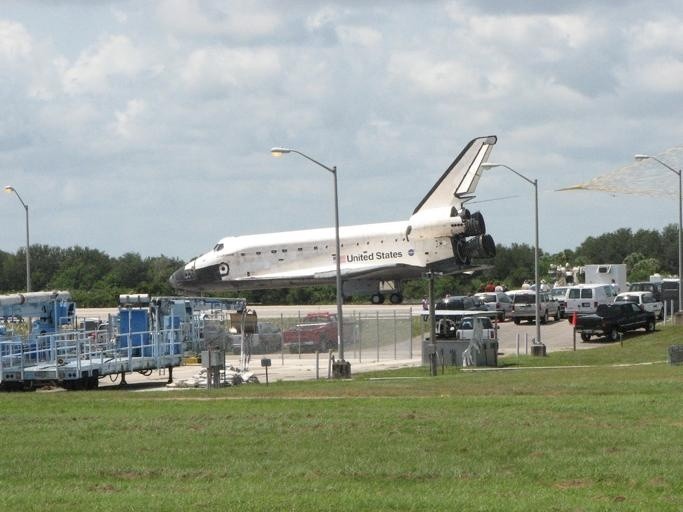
[421,294,429,323]
[610,278,620,297]
[521,278,550,291]
[494,282,503,292]
[477,282,485,292]
[485,280,495,292]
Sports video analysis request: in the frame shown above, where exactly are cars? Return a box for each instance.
[230,320,281,355]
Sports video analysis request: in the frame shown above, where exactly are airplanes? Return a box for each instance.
[166,132,502,306]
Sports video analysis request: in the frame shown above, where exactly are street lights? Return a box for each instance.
[478,159,547,358]
[634,154,683,316]
[268,146,352,379]
[2,185,33,338]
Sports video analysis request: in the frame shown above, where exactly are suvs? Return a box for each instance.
[421,277,679,343]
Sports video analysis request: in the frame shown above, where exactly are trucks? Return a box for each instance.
[282,311,361,354]
